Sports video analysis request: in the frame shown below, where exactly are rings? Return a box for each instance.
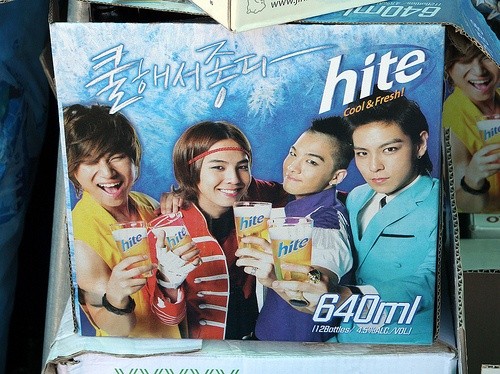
[289,291,309,307]
[307,267,320,284]
[250,266,257,275]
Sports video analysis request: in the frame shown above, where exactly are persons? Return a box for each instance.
[63,104,188,339]
[273,83,439,344]
[144,120,349,343]
[235,117,354,343]
[442,16,500,213]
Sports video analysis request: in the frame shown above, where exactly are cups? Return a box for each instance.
[147,213,202,269]
[109,221,153,278]
[475,114,500,164]
[267,217,313,281]
[233,201,272,259]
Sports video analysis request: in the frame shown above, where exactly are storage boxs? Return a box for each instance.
[43,0,500,374]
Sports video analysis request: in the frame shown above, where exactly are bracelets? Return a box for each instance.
[102,292,136,315]
[460,176,491,194]
